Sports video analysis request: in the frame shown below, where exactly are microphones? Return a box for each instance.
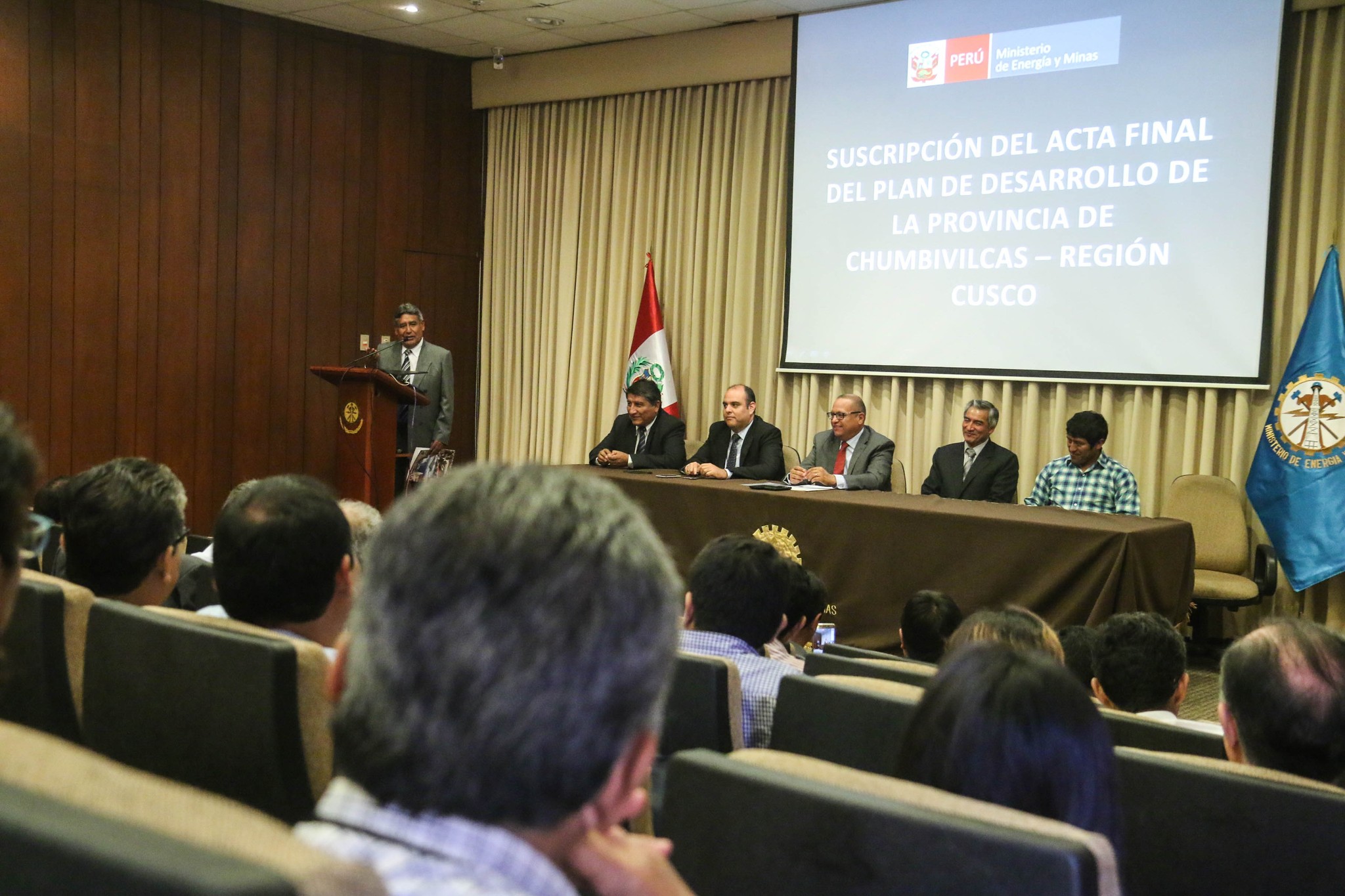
[346,337,408,366]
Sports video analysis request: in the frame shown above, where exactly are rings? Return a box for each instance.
[815,473,817,477]
[687,470,690,471]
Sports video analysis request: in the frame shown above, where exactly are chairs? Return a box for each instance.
[1,441,1344,896]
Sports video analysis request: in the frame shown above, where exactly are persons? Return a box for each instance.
[679,384,786,481]
[920,399,1019,505]
[779,393,896,493]
[589,377,687,468]
[1023,410,1141,518]
[0,396,1345,896]
[364,304,454,494]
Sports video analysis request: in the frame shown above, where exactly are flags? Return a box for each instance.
[1244,245,1345,591]
[618,251,678,416]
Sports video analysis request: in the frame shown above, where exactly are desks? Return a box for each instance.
[549,465,1194,656]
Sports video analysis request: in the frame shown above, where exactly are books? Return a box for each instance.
[407,446,454,482]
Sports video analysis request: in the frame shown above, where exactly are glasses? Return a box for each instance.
[827,411,862,420]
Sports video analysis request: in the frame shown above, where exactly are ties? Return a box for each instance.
[400,348,410,425]
[833,441,849,475]
[635,427,647,455]
[963,448,976,481]
[725,434,741,470]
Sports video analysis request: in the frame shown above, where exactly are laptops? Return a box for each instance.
[384,369,429,397]
[812,623,836,654]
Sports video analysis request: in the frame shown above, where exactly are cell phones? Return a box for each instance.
[749,485,792,491]
[656,474,681,478]
